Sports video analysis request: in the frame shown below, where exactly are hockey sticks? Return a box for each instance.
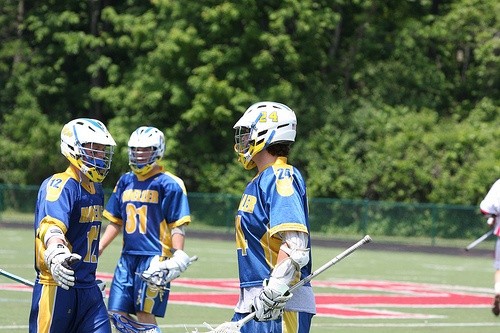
[465,229,494,252]
[0,268,161,333]
[202,234,372,333]
[134,255,199,287]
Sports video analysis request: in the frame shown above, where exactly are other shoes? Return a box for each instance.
[492,294,500,316]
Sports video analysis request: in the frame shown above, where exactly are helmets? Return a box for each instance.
[128,126,165,177]
[233,102,297,171]
[61,118,116,183]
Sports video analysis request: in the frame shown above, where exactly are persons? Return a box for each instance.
[99,126,191,333]
[480,178,500,317]
[29,118,113,333]
[230,101,316,333]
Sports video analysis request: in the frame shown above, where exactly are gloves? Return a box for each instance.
[248,278,293,322]
[44,243,82,290]
[142,249,198,285]
